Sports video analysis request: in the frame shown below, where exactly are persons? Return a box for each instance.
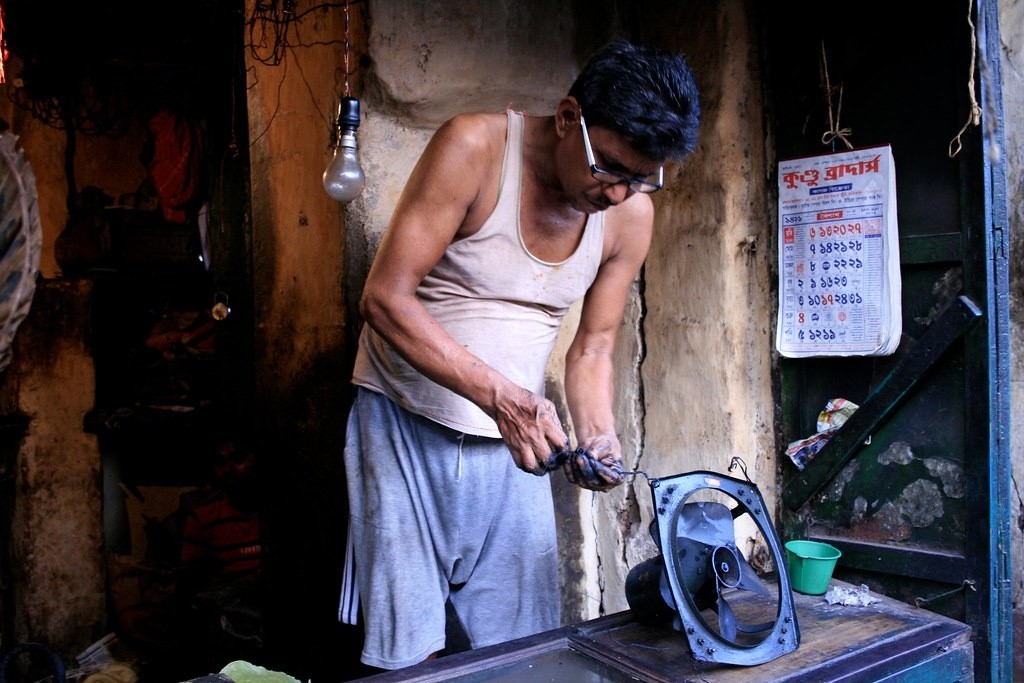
[70,179,189,266]
[338,40,700,673]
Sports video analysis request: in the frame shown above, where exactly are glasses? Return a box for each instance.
[578,107,664,194]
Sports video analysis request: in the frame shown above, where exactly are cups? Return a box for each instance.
[785,540,843,595]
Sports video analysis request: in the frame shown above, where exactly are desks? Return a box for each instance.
[356,573,974,683]
